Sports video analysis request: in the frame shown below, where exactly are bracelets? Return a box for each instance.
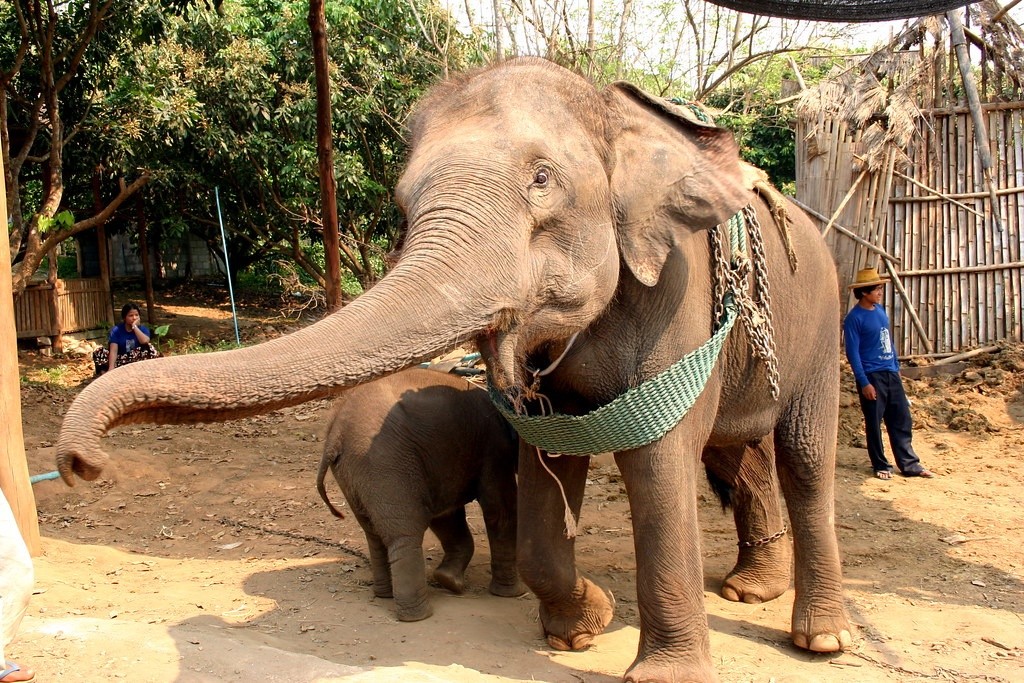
[132,324,137,329]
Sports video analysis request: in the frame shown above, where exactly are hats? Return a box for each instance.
[847,268,891,289]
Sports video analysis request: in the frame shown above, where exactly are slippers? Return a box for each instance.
[914,467,934,478]
[876,469,893,480]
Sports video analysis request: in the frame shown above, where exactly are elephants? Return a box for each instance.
[57,54,856,683]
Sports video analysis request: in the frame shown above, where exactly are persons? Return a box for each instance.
[843,267,935,479]
[0,486,35,683]
[93,302,158,380]
